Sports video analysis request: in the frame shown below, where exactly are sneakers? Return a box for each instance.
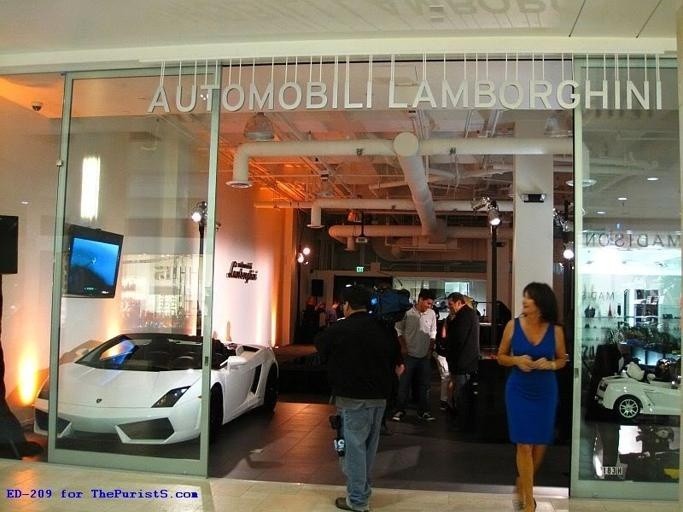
[333,495,370,512]
[392,409,407,422]
[417,411,436,421]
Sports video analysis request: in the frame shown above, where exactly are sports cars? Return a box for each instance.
[33,333,281,447]
[595,357,681,420]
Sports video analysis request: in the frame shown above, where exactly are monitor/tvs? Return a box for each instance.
[66,224,124,298]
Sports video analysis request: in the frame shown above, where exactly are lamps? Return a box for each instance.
[562,239,575,261]
[242,113,275,141]
[486,199,502,228]
[543,109,573,139]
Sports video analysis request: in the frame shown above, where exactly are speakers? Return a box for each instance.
[0,215,18,273]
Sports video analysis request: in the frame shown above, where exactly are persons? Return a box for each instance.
[303,285,480,512]
[498,283,566,512]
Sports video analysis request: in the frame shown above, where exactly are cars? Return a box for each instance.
[433,296,450,309]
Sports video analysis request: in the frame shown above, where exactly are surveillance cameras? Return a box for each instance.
[32,103,42,112]
